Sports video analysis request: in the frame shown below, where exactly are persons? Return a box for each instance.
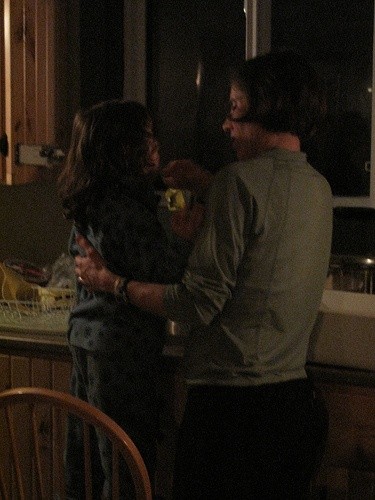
[56,100,206,500]
[74,55,332,500]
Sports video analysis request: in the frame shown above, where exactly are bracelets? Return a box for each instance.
[114,276,131,308]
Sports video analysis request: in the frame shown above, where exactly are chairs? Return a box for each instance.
[0,387,152,500]
